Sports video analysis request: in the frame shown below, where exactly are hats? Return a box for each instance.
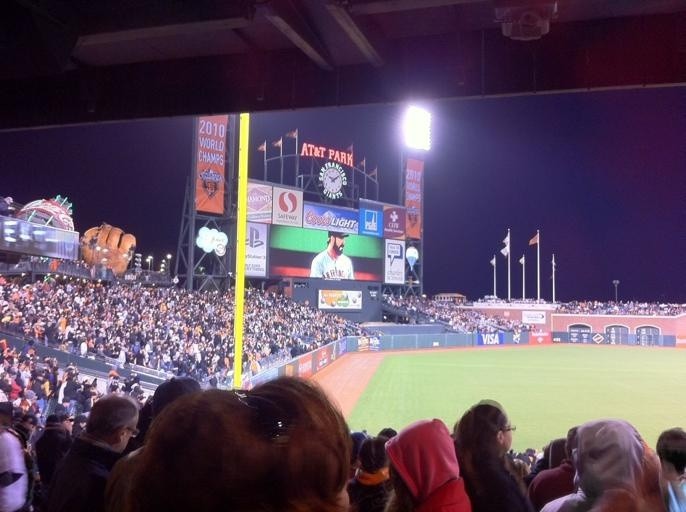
[328,231,349,238]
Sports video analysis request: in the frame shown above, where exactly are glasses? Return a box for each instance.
[117,425,140,437]
[502,424,517,432]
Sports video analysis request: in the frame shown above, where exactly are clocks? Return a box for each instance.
[323,167,343,193]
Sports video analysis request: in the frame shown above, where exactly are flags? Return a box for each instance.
[287,128,295,138]
[489,256,494,267]
[502,233,508,249]
[500,245,509,256]
[518,257,524,266]
[367,167,376,176]
[256,142,264,152]
[359,159,364,167]
[272,138,280,148]
[528,234,537,246]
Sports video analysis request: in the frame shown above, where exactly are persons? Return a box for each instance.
[1,274,366,389]
[382,294,548,334]
[306,231,356,279]
[2,390,686,512]
[554,300,686,315]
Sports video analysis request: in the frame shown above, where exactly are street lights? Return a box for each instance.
[95,246,173,278]
[612,279,620,304]
[400,105,432,298]
